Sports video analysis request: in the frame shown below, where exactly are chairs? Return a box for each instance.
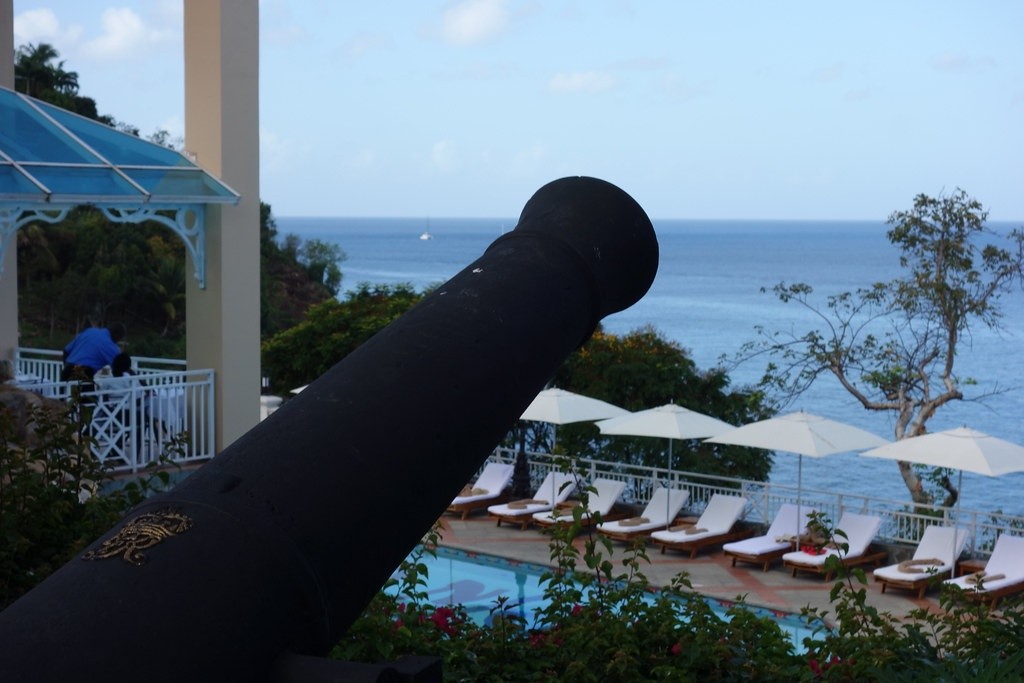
[651,493,755,560]
[942,533,1024,613]
[723,504,817,573]
[532,477,630,535]
[488,471,581,531]
[782,511,888,583]
[449,463,516,520]
[596,487,691,550]
[873,526,969,601]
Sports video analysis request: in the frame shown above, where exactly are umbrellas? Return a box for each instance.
[518,387,1024,580]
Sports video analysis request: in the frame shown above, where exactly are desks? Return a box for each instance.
[144,390,185,440]
[675,516,698,526]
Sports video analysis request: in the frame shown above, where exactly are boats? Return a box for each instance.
[420,231,434,240]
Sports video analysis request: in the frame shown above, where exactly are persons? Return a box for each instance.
[61,322,158,465]
